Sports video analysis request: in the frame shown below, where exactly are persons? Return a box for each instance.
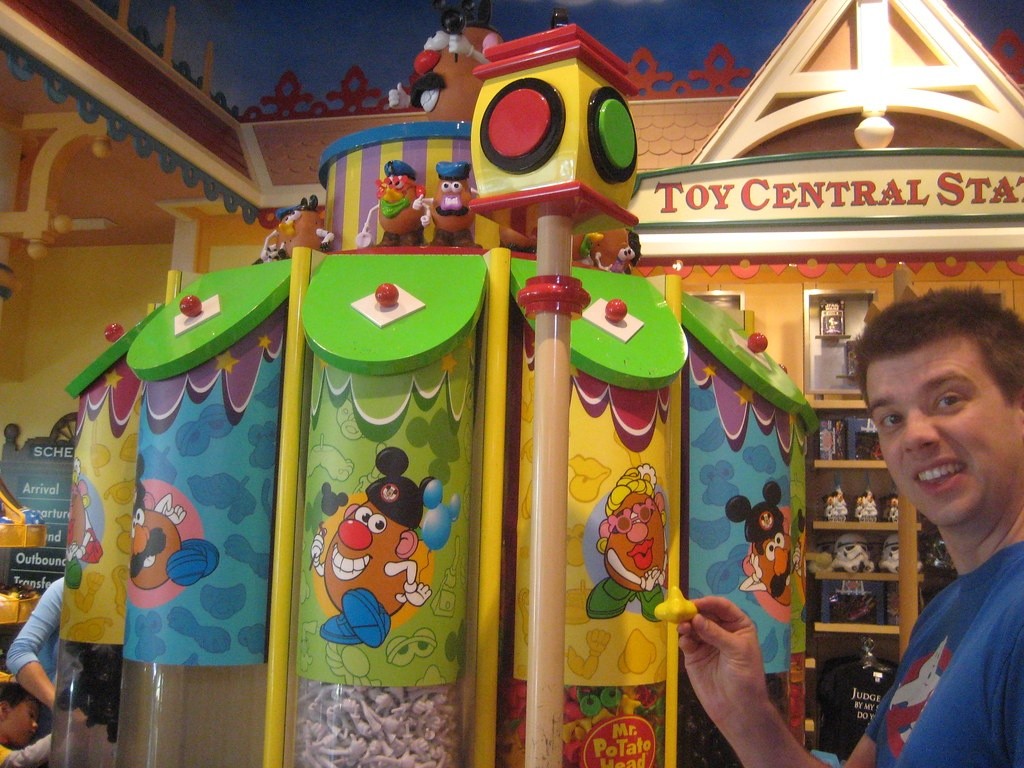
[6,577,65,745]
[677,284,1024,768]
[0,672,53,768]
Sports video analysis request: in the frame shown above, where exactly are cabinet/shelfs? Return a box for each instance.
[802,398,924,661]
[0,480,48,685]
